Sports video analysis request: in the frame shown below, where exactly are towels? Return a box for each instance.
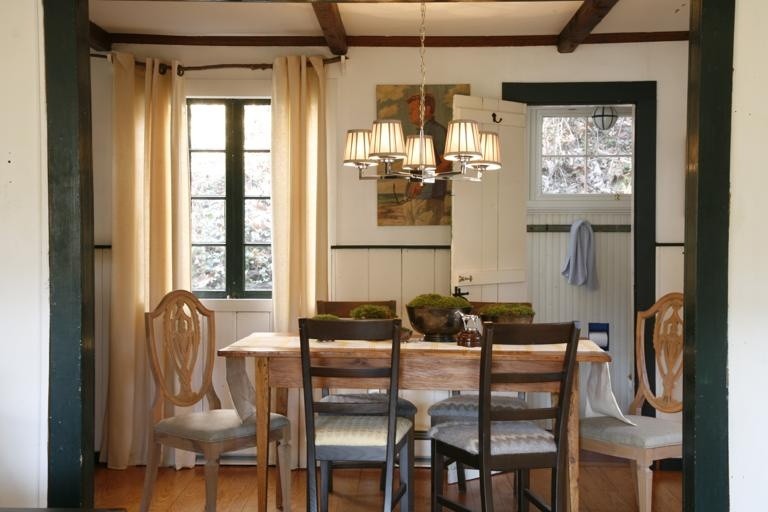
[560,219,601,291]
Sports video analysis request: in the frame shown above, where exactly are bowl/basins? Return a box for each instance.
[457,330,482,347]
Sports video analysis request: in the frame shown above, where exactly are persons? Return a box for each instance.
[401,94,451,226]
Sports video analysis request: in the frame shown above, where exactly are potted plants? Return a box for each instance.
[406,294,473,341]
[479,303,536,326]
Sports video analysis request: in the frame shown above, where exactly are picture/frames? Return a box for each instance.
[376,83,471,225]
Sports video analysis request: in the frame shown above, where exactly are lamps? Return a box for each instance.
[593,107,618,131]
[342,2,502,187]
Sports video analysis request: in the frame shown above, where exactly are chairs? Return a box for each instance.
[578,292,683,512]
[298,318,414,512]
[315,299,417,492]
[141,290,291,512]
[430,321,581,512]
[428,301,534,495]
